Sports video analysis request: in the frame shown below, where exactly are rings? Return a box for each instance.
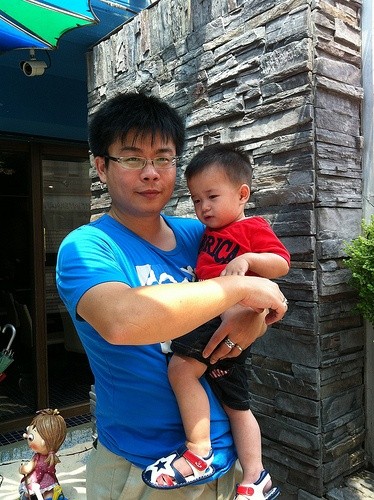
[236,345,243,353]
[282,297,287,304]
[225,338,235,348]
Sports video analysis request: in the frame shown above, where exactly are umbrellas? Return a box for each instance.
[0,324,16,375]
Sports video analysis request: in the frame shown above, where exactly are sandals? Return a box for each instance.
[232,470,281,500]
[141,444,215,489]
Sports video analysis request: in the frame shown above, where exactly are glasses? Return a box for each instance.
[103,155,179,169]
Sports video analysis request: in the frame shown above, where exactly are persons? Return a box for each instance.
[55,92,288,500]
[17,408,67,500]
[141,143,291,500]
